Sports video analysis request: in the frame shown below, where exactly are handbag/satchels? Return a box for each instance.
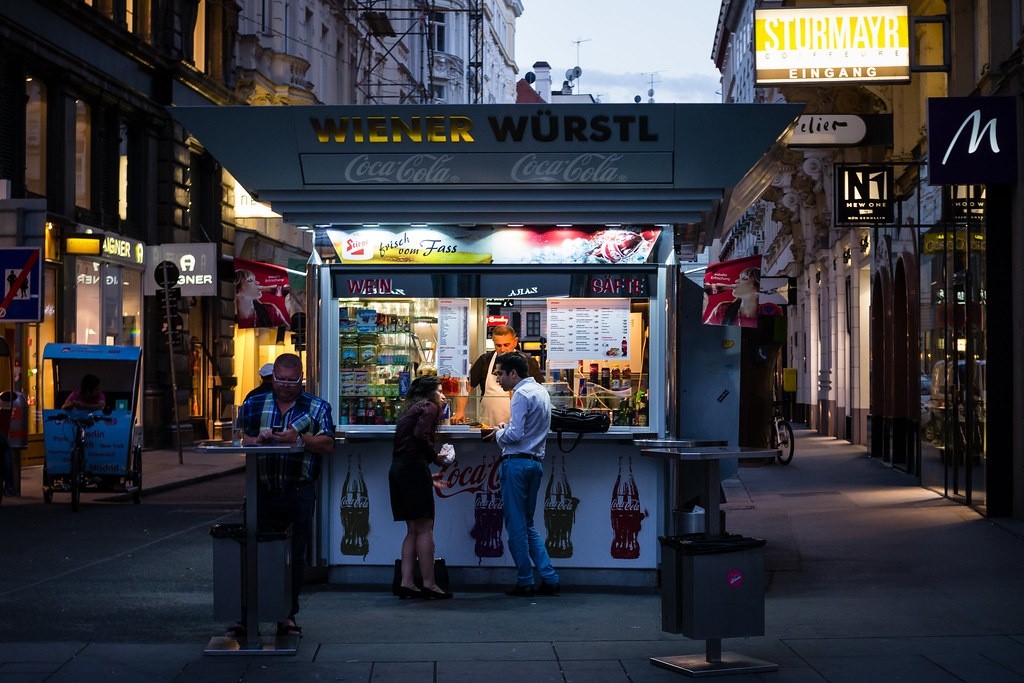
[550,407,611,434]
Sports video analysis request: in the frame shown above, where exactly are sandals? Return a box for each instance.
[280,614,304,639]
[224,621,261,638]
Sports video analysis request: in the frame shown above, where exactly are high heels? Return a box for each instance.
[398,586,431,600]
[420,587,453,599]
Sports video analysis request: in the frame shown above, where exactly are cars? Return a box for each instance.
[919,373,939,443]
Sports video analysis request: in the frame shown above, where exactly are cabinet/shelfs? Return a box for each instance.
[339,297,418,425]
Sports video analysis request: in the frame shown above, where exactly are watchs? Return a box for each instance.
[295,431,303,445]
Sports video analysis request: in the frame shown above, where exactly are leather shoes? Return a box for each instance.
[537,582,561,596]
[506,583,537,597]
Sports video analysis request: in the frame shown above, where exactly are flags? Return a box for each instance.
[700,253,785,329]
[231,254,293,333]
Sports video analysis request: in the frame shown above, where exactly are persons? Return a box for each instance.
[235,269,292,330]
[479,348,562,599]
[223,351,337,641]
[385,373,455,602]
[242,362,276,403]
[450,324,549,428]
[704,266,782,328]
[60,373,107,413]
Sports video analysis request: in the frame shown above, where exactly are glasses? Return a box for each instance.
[271,372,303,387]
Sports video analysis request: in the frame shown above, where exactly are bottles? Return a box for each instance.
[705,283,738,297]
[472,452,503,557]
[543,453,574,558]
[609,454,642,560]
[339,394,406,424]
[574,357,648,427]
[621,336,627,356]
[258,284,289,297]
[341,448,369,557]
[583,228,655,264]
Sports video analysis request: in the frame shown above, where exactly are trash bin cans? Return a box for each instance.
[635,436,730,535]
[638,444,784,677]
[198,443,310,655]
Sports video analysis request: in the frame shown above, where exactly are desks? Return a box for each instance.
[638,441,786,677]
[629,436,731,634]
[202,438,306,656]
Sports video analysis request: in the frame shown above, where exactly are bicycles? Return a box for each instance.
[760,397,794,466]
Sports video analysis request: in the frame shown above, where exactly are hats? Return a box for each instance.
[259,362,274,377]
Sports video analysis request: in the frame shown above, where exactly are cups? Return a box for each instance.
[231,402,244,448]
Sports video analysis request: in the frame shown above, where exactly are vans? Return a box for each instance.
[931,358,986,451]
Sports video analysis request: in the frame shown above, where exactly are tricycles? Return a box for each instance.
[38,341,145,506]
